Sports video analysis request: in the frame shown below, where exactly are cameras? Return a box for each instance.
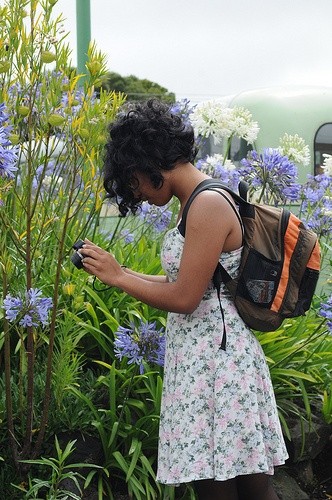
[71,239,90,269]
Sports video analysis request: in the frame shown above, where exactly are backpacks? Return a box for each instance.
[177,177,321,351]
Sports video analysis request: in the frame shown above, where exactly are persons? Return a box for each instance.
[79,99,288,500]
